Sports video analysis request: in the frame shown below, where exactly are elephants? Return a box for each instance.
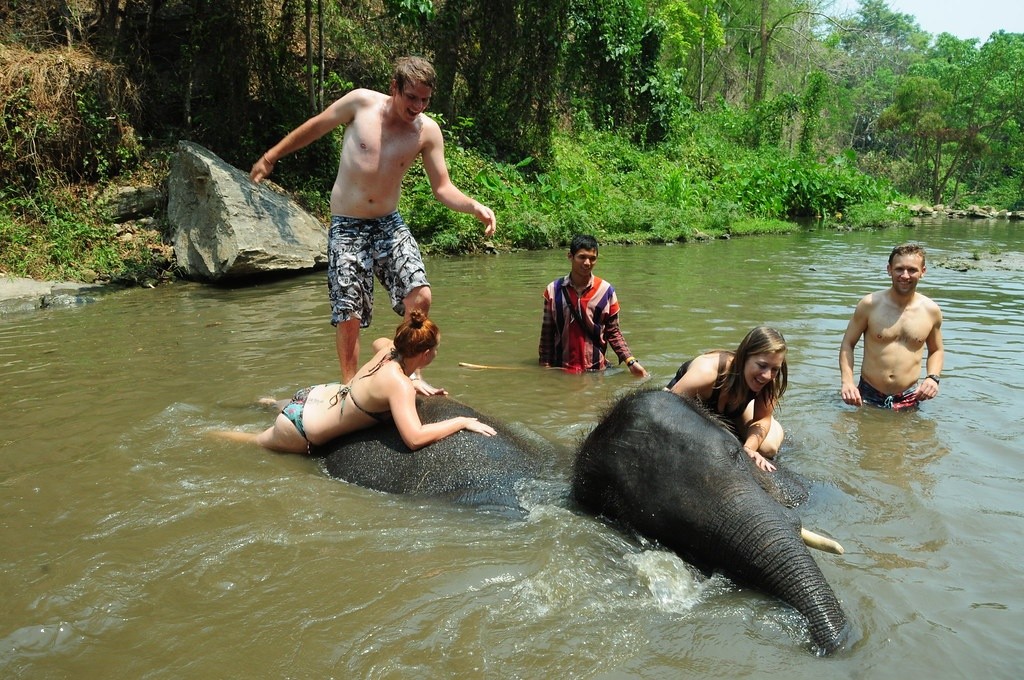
[213,385,864,658]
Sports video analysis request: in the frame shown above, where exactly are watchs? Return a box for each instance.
[925,374,940,384]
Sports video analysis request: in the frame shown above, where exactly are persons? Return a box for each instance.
[206,309,498,454]
[663,327,788,473]
[251,57,496,382]
[839,245,944,411]
[539,235,648,378]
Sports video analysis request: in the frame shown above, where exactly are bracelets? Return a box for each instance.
[627,360,638,367]
[263,152,272,164]
